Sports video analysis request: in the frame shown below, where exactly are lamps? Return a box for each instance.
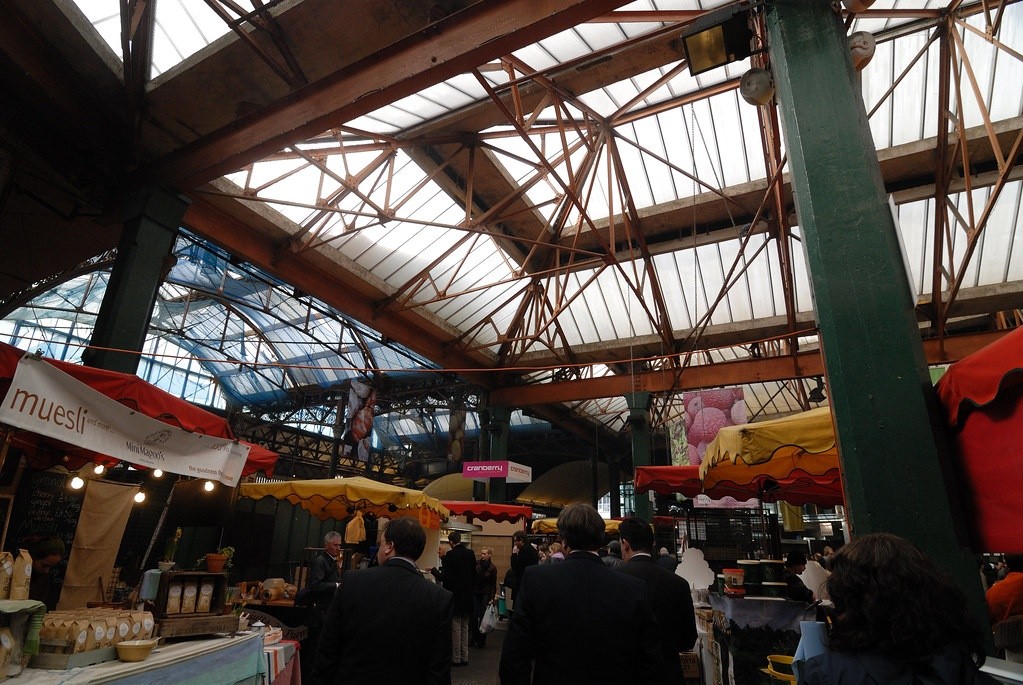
[679,0,770,77]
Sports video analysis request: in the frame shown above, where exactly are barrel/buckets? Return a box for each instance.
[722,569,745,595]
[717,574,738,595]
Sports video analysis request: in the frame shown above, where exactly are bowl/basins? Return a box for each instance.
[115,639,157,661]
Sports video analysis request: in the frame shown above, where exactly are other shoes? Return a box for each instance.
[461,662,468,665]
[451,663,460,666]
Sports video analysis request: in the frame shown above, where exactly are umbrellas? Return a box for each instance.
[241,472,451,560]
[440,498,533,525]
[931,318,1022,554]
[691,409,840,490]
[631,454,843,560]
[530,516,623,534]
[0,340,279,481]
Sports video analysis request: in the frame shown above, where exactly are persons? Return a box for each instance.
[597,528,626,568]
[978,552,1023,664]
[425,531,477,666]
[468,545,497,649]
[536,537,565,564]
[498,503,674,685]
[307,515,453,685]
[611,516,698,685]
[657,545,678,573]
[306,528,344,668]
[777,544,839,605]
[796,531,986,685]
[28,535,64,600]
[510,530,538,610]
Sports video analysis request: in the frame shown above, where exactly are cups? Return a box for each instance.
[251,619,265,639]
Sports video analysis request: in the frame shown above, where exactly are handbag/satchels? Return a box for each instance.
[479,603,498,634]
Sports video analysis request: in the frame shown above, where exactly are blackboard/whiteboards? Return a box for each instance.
[3,467,89,611]
